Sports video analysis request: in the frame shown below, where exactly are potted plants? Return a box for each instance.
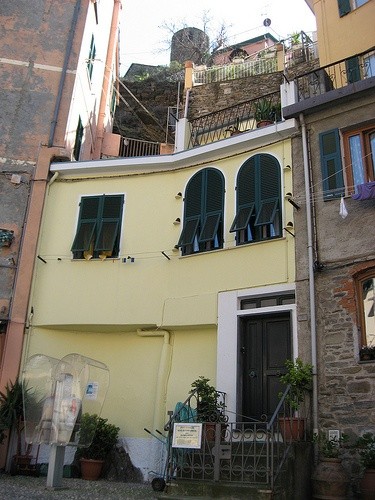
[225,117,250,136]
[188,375,227,442]
[351,432,375,500]
[277,358,314,442]
[75,412,120,481]
[290,32,302,50]
[253,98,281,128]
[310,429,351,500]
[0,376,47,465]
[231,55,244,64]
